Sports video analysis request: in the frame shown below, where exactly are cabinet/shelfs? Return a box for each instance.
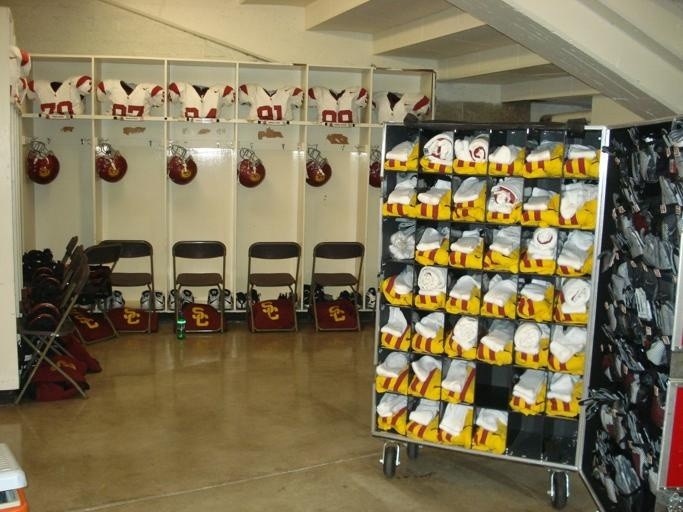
[369,117,608,471]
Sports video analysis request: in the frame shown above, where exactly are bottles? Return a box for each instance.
[175,311,187,340]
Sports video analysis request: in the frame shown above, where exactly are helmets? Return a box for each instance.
[28,141,60,183]
[306,148,331,187]
[239,149,265,187]
[369,150,381,187]
[168,144,197,184]
[95,144,127,182]
